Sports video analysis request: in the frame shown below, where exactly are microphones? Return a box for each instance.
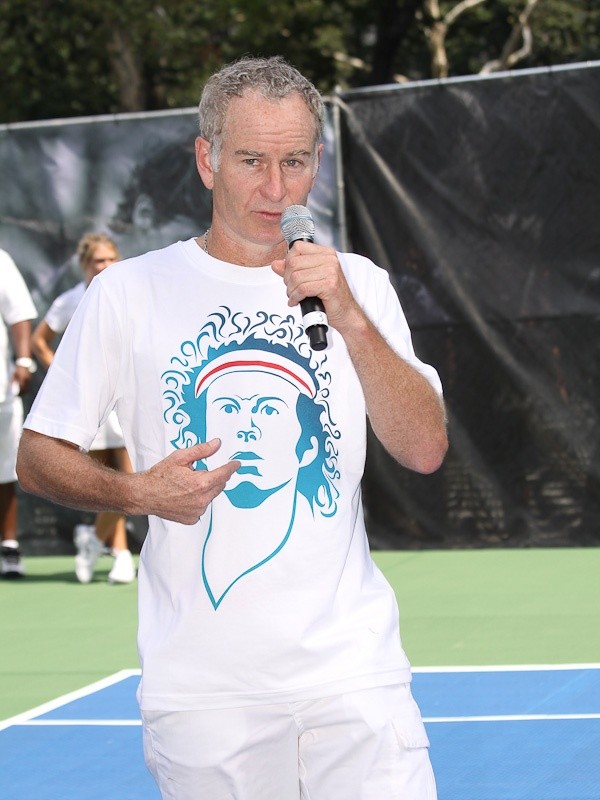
[281,204,330,351]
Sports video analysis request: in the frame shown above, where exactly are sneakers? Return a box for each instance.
[107,549,135,582]
[0,547,23,577]
[74,523,104,584]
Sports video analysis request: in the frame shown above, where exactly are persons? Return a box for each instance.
[16,58,447,800]
[0,234,135,584]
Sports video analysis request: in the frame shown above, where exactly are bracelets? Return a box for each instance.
[15,357,38,372]
